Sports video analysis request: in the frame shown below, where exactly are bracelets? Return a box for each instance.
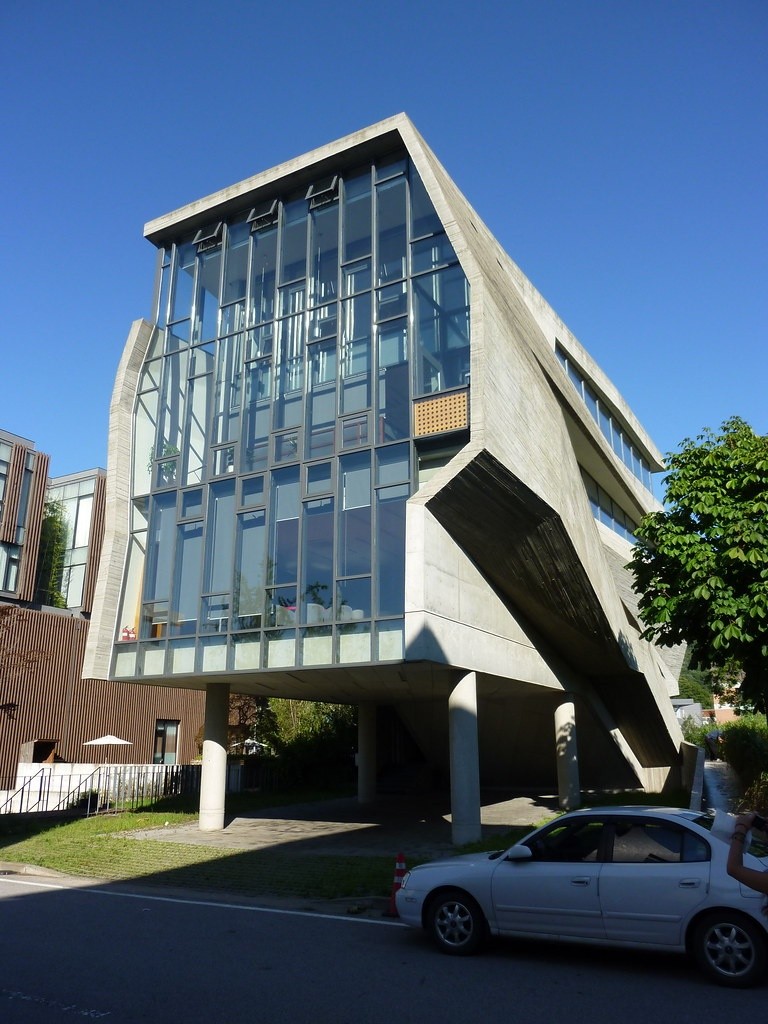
[735,823,749,833]
[731,832,746,843]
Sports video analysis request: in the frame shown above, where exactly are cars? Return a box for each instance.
[395,803,767,990]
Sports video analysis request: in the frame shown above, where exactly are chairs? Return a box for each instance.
[151,608,185,638]
[277,605,364,626]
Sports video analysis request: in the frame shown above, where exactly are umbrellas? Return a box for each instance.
[83,735,134,764]
[232,739,267,754]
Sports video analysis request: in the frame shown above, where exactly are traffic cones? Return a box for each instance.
[382,851,408,918]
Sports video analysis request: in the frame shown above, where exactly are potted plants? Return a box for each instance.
[148,439,180,488]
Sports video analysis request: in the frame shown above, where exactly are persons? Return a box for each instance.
[727,811,768,895]
